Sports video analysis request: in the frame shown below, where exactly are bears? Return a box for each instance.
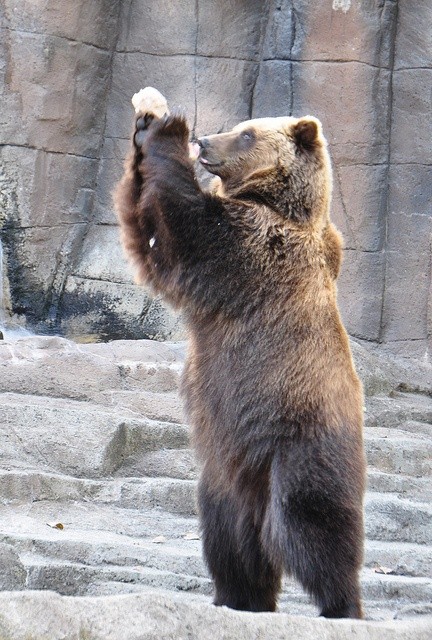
[110,110,366,618]
